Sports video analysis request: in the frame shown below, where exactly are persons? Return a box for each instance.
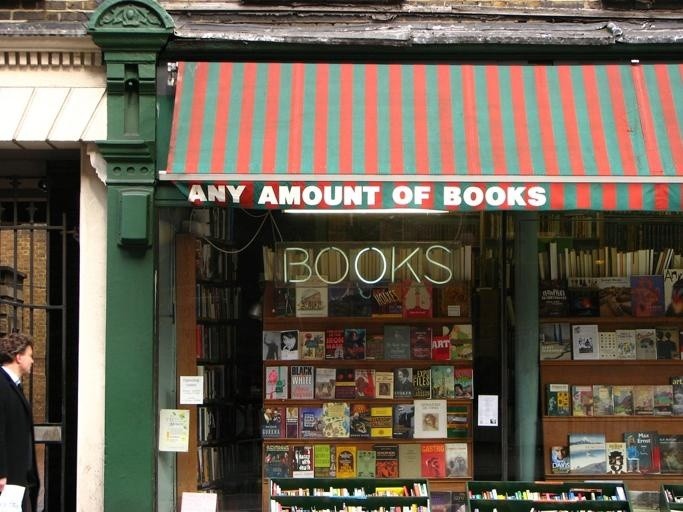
[0,333,41,512]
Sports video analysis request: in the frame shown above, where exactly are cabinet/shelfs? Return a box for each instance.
[175,219,473,512]
[502,212,682,512]
[268,477,431,512]
[466,480,633,512]
[658,484,683,511]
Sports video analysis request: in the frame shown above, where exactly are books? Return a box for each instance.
[175,204,683,512]
